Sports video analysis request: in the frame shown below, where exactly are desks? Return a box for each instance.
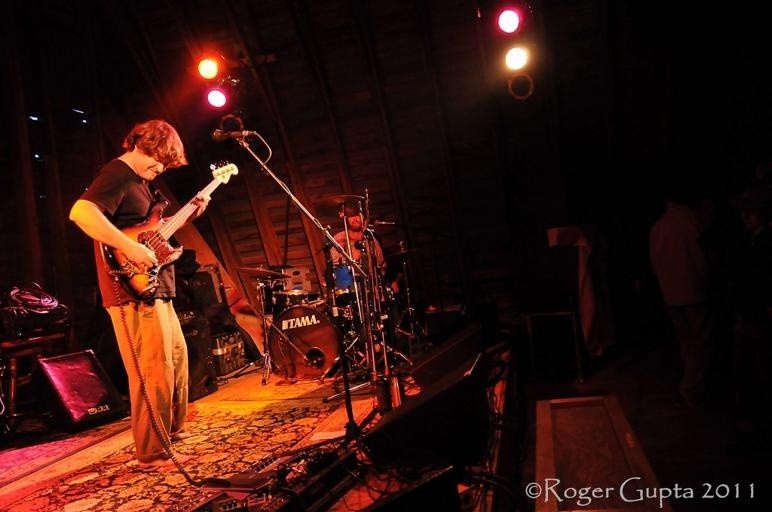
[0,331,66,413]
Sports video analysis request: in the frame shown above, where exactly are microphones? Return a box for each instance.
[306,347,325,369]
[210,127,256,142]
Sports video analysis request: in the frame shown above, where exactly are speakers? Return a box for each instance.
[27,348,127,435]
[359,349,491,481]
[357,462,462,512]
[171,269,226,403]
[409,326,498,387]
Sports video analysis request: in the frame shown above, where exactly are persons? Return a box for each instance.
[69,120,212,467]
[330,204,387,276]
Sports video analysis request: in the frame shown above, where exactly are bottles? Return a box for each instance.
[375,374,391,414]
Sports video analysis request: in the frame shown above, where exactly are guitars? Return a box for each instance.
[99,161,239,302]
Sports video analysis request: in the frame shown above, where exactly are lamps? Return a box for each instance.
[193,50,242,114]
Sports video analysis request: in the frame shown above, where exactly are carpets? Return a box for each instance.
[1,395,344,510]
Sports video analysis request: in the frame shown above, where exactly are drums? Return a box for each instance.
[268,300,355,380]
[332,263,362,296]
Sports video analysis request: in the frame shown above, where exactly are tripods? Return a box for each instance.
[317,188,413,449]
[234,280,281,385]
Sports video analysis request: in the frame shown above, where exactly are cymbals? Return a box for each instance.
[312,194,366,216]
[387,249,417,258]
[272,289,320,300]
[239,268,291,281]
